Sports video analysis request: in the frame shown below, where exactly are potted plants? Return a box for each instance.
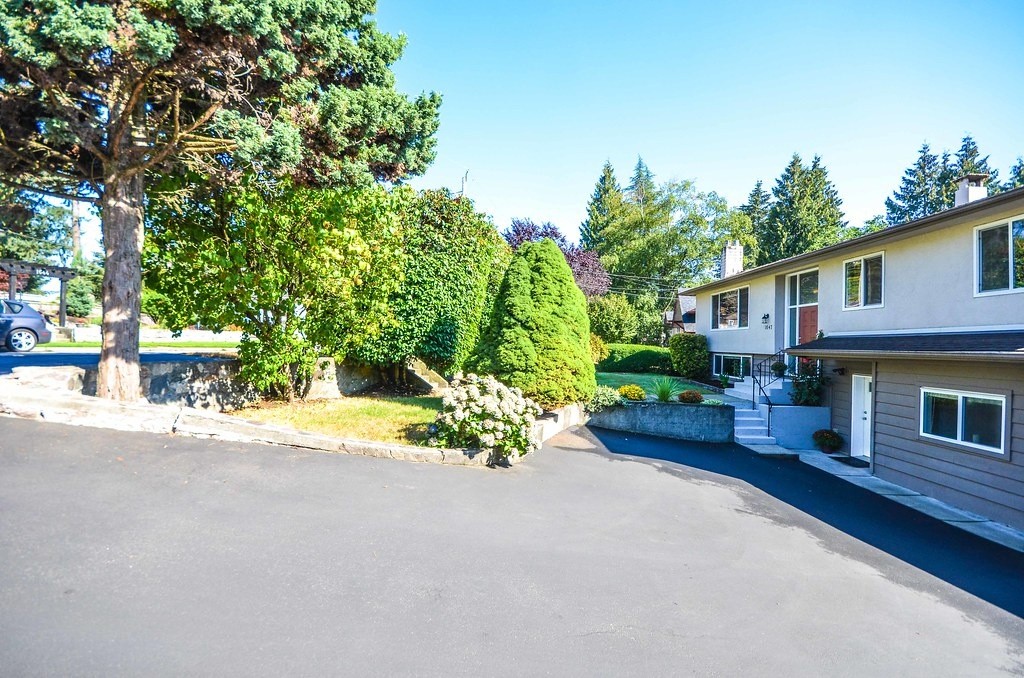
[719,371,729,387]
[771,362,787,377]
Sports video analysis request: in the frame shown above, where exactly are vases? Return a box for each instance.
[821,445,834,453]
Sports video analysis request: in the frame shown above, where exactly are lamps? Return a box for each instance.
[762,314,769,324]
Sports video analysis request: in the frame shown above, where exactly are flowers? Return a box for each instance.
[813,428,844,448]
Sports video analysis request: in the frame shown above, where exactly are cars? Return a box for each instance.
[0,299,52,353]
